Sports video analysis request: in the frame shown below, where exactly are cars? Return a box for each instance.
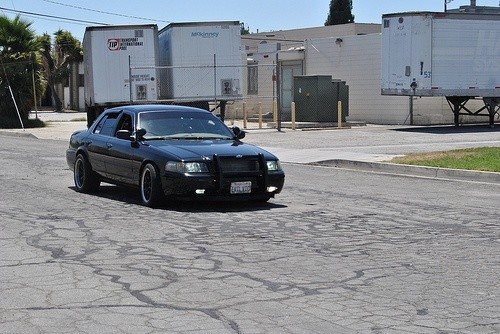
[67,105,285,207]
